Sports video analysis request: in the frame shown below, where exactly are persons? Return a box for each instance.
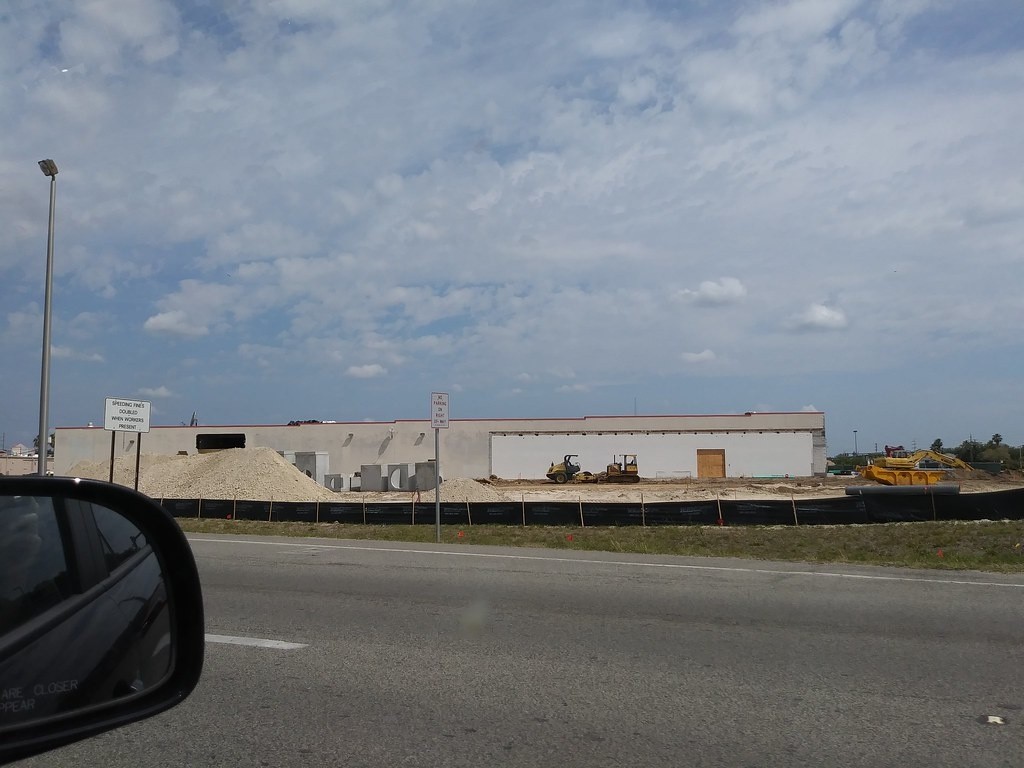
[0,494,45,610]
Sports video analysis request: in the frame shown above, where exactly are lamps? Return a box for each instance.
[349,433,354,438]
[130,440,134,444]
[420,433,425,437]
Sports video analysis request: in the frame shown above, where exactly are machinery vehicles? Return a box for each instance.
[599,453,641,483]
[545,454,598,484]
[854,444,976,486]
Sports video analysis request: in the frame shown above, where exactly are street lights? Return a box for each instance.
[853,430,858,454]
[37,160,66,473]
